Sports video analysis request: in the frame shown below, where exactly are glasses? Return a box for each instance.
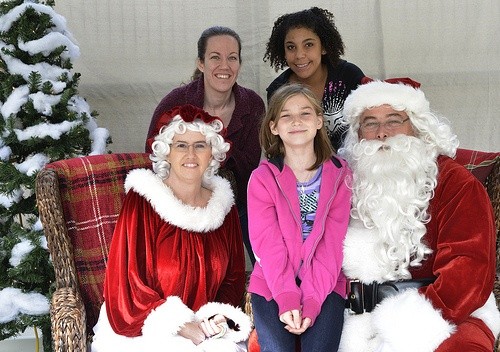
[359,118,409,131]
[171,141,210,153]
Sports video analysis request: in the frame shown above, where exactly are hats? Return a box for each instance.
[148,104,233,166]
[341,76,431,121]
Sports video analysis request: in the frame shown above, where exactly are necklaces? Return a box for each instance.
[288,159,315,207]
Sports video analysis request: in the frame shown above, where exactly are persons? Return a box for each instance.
[246,85,354,352]
[265,6,368,157]
[247,77,500,352]
[145,26,266,269]
[91,104,253,352]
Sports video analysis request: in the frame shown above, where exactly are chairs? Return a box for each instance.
[452,148,500,352]
[36,153,255,352]
